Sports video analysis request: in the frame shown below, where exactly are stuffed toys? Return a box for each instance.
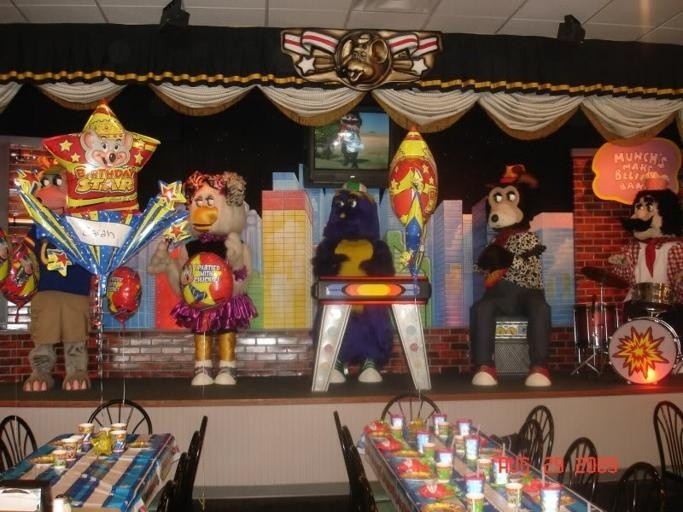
[469,166,552,388]
[21,162,91,393]
[611,173,683,320]
[318,179,392,384]
[148,172,252,386]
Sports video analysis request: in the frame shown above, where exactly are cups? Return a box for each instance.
[50,422,129,470]
[388,411,561,512]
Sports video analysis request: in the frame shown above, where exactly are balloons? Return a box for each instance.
[107,266,142,323]
[0,232,39,324]
[41,99,161,213]
[386,127,439,276]
[183,252,234,313]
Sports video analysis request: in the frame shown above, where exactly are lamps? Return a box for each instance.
[555,14,588,54]
[154,0,190,39]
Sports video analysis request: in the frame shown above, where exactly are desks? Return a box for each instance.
[1,433,176,512]
[359,419,605,511]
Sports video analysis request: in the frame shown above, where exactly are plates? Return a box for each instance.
[367,432,392,440]
[29,457,55,468]
[531,494,575,507]
[125,441,152,451]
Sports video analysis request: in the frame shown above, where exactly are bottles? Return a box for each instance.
[53,493,69,512]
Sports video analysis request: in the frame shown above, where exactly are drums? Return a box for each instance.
[573,303,616,349]
[634,281,674,309]
[608,316,681,384]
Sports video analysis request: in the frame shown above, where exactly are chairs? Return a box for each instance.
[328,407,396,511]
[379,393,443,423]
[489,396,682,511]
[158,416,208,510]
[0,413,36,473]
[0,479,57,512]
[86,396,153,434]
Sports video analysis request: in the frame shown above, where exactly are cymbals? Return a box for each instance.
[581,267,627,288]
